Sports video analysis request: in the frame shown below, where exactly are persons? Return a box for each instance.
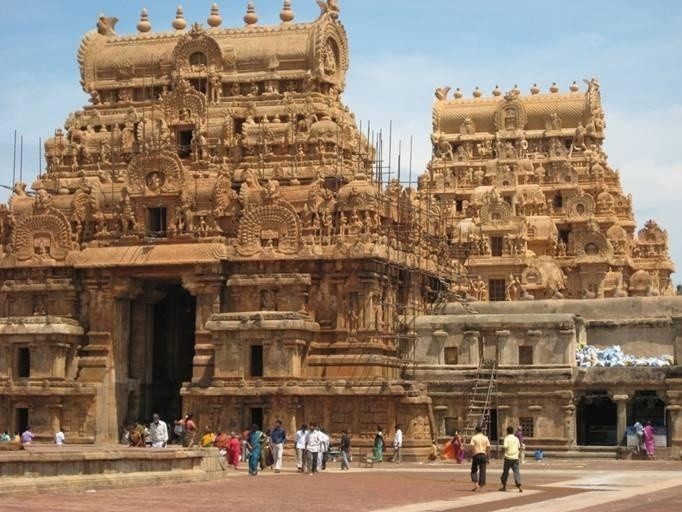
[121,410,353,475]
[54,428,65,445]
[534,448,545,460]
[450,431,465,463]
[641,421,656,457]
[0,430,11,440]
[515,425,526,463]
[11,431,21,442]
[392,424,402,463]
[468,425,490,492]
[371,425,384,462]
[633,418,644,453]
[21,426,35,442]
[427,437,438,461]
[499,426,523,493]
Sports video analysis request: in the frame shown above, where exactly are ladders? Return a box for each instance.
[462,361,497,445]
